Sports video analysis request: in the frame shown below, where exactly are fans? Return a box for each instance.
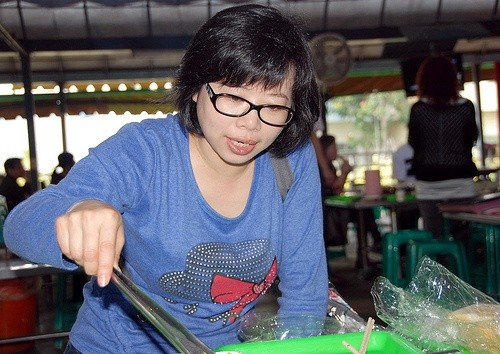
[309,33,352,85]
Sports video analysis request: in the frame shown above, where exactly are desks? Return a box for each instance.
[325,187,422,276]
[442,198,500,299]
[0,258,96,346]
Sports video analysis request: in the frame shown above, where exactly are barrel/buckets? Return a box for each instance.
[0,275,42,354]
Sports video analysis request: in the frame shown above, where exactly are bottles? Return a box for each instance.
[346,222,357,244]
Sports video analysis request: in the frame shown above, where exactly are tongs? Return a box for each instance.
[103,269,214,354]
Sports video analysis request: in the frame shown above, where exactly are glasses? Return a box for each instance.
[205,83,295,127]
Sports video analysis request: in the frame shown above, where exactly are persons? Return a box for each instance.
[0,0,328,354]
[392,58,480,240]
[321,135,388,268]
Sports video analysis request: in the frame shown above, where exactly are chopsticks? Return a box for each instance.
[358,317,375,354]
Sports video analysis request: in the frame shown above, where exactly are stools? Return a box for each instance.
[383,230,473,300]
